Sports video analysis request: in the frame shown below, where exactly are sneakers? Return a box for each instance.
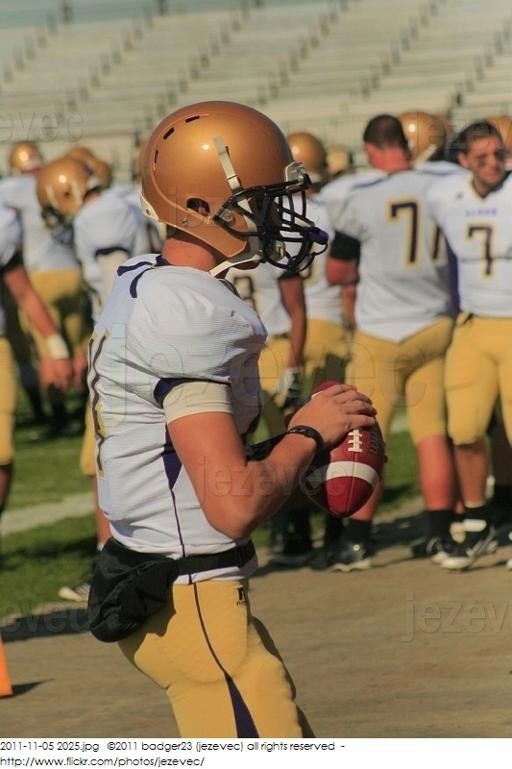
[266,500,511,575]
[59,559,98,602]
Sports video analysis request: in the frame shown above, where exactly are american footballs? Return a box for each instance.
[288,380,385,516]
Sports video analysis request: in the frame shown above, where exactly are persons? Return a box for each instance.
[228,110,512,571]
[0,140,163,609]
[82,100,378,738]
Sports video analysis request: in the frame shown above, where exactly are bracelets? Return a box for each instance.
[286,426,324,455]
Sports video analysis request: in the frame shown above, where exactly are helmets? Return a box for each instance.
[485,115,512,173]
[139,101,329,283]
[286,132,328,186]
[398,111,447,162]
[8,139,112,247]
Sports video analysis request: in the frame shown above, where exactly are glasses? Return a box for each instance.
[476,148,504,166]
